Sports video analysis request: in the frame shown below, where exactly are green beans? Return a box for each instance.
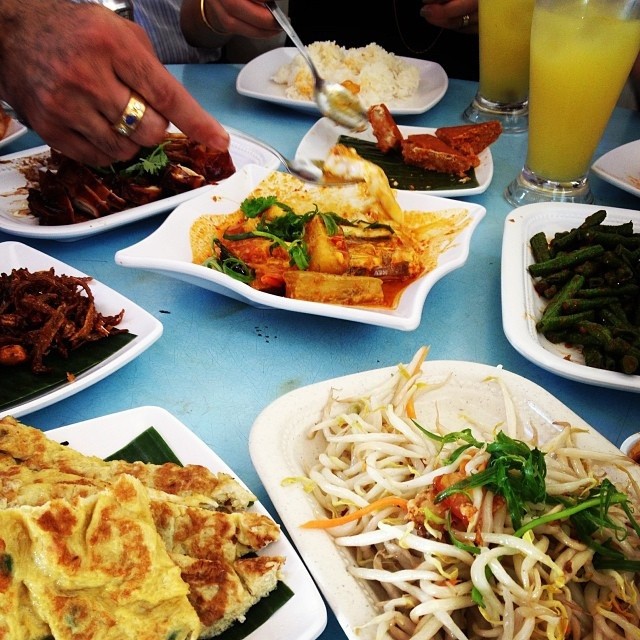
[528,210,640,374]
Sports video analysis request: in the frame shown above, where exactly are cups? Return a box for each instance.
[504,1,639,209]
[461,1,534,134]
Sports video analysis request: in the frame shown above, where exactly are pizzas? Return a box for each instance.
[0,414,284,640]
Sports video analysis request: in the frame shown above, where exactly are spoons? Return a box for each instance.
[263,0,371,131]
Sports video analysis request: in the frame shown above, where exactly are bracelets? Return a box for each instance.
[200,0,233,40]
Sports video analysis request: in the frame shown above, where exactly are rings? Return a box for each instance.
[113,92,148,137]
[462,15,469,27]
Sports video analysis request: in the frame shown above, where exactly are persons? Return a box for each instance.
[127,0,479,79]
[1,0,229,170]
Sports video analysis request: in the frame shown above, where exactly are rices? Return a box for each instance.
[273,40,425,107]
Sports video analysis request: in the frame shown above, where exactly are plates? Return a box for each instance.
[0,240,165,421]
[501,201,640,394]
[619,431,640,466]
[236,46,449,117]
[0,122,283,243]
[34,403,329,640]
[590,141,640,198]
[114,161,487,332]
[0,117,27,147]
[290,117,494,199]
[246,359,640,640]
